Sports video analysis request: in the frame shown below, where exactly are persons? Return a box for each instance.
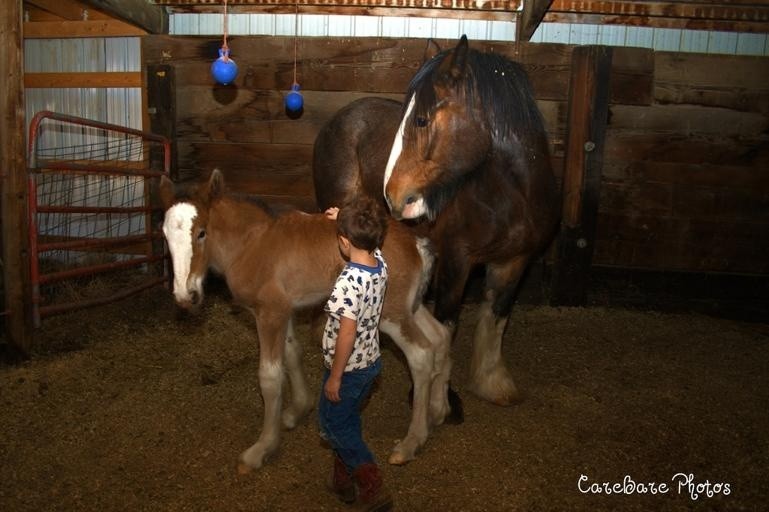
[321,198,396,512]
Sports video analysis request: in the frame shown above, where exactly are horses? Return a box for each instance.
[312,33,559,426]
[158,168,453,476]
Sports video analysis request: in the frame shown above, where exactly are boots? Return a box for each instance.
[331,455,357,504]
[354,464,395,510]
